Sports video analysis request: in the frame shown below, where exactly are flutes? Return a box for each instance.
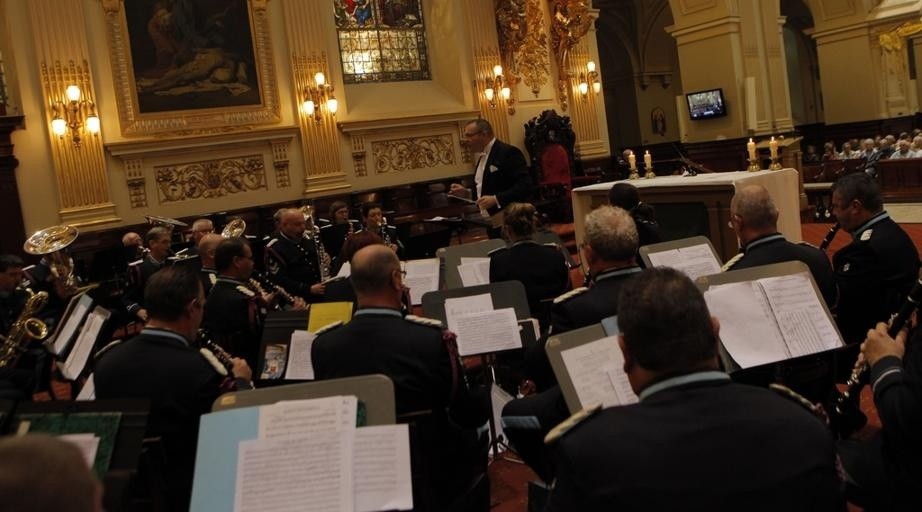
[832,277,921,416]
[254,270,298,303]
[820,221,840,252]
[198,329,238,375]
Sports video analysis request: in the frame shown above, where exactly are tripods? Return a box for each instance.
[480,354,525,468]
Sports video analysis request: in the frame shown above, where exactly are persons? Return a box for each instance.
[448,120,536,235]
[491,183,679,485]
[727,171,922,442]
[527,266,849,512]
[837,323,921,512]
[803,128,921,161]
[1,169,492,512]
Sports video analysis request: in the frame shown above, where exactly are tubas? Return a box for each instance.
[24,226,81,299]
[140,212,186,247]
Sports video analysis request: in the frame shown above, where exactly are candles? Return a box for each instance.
[643,150,651,167]
[746,138,756,160]
[628,151,636,168]
[769,136,778,157]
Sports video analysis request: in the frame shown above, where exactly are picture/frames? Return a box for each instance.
[101,0,283,138]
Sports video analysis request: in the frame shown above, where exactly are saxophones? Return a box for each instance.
[218,218,243,254]
[300,230,331,280]
[1,278,49,371]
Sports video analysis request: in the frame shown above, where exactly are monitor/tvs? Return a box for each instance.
[685,87,727,121]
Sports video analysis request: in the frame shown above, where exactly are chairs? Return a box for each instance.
[311,179,482,247]
[522,107,606,226]
[801,155,882,218]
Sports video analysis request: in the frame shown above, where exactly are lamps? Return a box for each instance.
[51,85,101,153]
[302,71,338,124]
[485,65,509,109]
[579,61,601,103]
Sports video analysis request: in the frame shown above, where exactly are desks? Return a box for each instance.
[572,167,802,264]
